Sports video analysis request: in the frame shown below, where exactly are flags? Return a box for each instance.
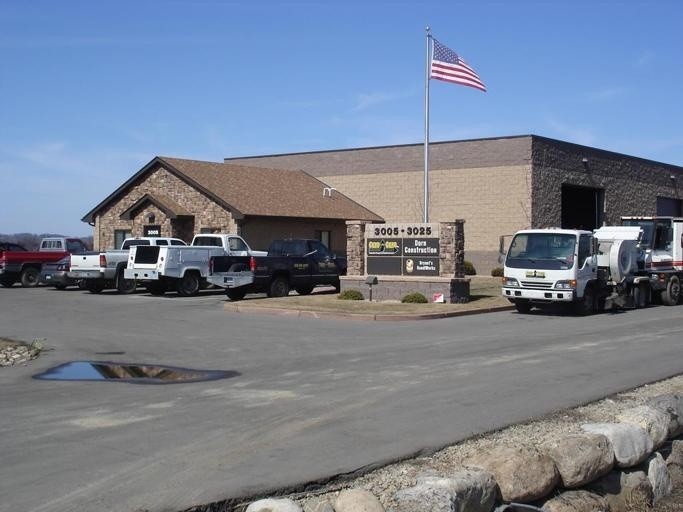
[430,36,487,92]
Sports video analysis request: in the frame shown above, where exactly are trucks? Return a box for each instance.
[499,215,683,313]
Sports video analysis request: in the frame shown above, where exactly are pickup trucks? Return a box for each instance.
[207,237,347,301]
[0,237,90,288]
[123,234,268,297]
[66,237,189,294]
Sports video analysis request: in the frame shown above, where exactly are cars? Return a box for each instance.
[0,243,29,252]
[39,251,105,290]
